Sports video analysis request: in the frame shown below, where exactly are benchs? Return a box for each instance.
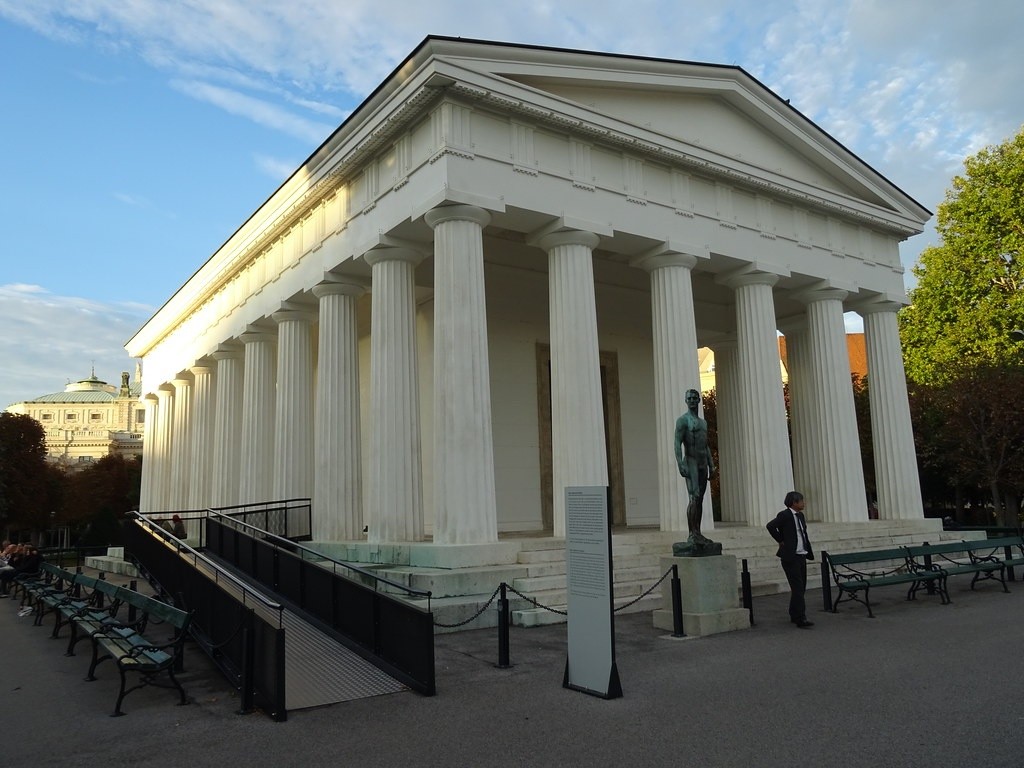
[821,537,1024,618]
[10,562,200,717]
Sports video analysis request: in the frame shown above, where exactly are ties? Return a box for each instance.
[795,513,807,551]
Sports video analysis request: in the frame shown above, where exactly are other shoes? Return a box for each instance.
[790,616,796,623]
[0,593,8,598]
[796,620,814,628]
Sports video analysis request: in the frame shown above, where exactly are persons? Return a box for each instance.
[767,491,815,628]
[0,539,45,597]
[150,514,186,539]
[673,390,715,546]
